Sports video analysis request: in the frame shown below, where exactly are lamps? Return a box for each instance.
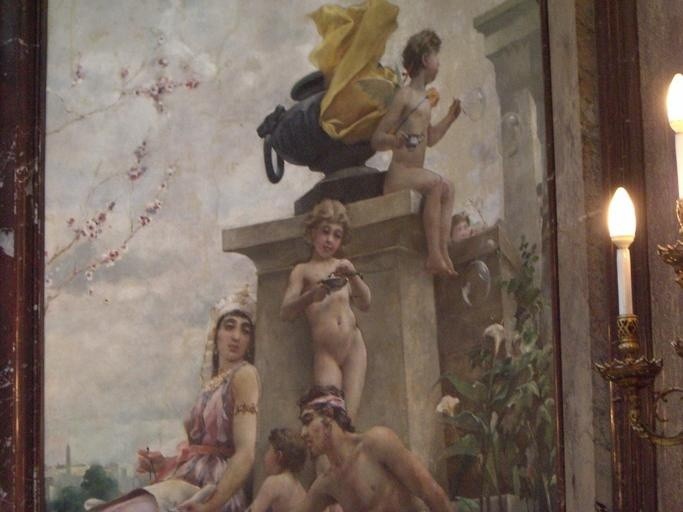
[592,72,682,447]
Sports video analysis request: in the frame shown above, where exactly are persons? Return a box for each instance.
[369,29,460,278]
[90,281,262,512]
[244,428,308,512]
[279,199,373,512]
[289,385,456,512]
[450,213,471,244]
